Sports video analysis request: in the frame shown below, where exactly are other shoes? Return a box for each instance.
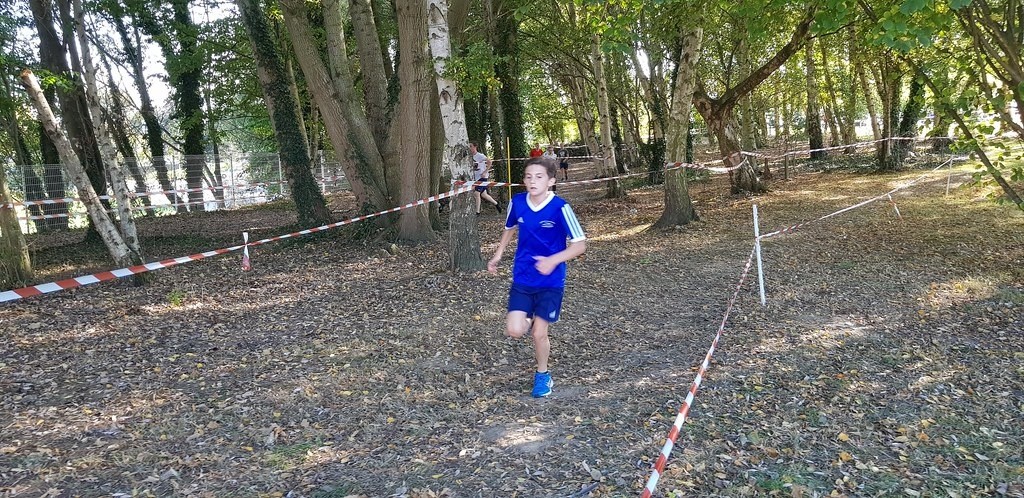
[566,177,568,180]
[561,178,564,181]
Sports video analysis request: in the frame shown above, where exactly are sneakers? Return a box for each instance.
[494,201,503,213]
[476,212,480,217]
[530,372,554,397]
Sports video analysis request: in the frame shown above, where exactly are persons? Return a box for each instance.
[529,142,544,159]
[546,146,557,195]
[470,140,503,216]
[487,157,587,398]
[558,143,569,181]
